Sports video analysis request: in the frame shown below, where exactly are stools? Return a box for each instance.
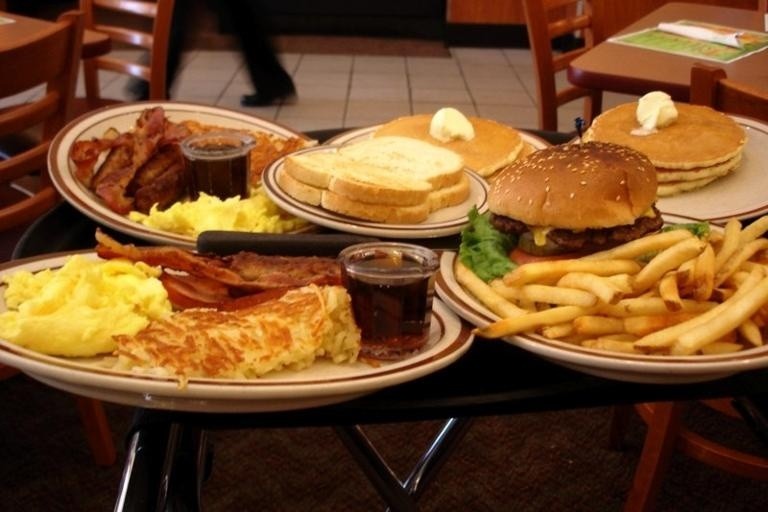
[626,401,767,512]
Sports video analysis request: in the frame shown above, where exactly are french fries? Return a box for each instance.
[466,215,767,363]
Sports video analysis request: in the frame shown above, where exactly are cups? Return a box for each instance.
[179,134,256,202]
[337,241,439,359]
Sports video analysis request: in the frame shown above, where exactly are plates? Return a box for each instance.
[434,213,768,382]
[322,125,555,147]
[47,99,322,248]
[262,144,490,238]
[569,114,768,225]
[1,245,476,412]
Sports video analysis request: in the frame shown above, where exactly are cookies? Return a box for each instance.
[582,98,749,198]
[364,113,538,185]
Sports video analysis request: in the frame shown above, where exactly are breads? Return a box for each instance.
[279,136,472,226]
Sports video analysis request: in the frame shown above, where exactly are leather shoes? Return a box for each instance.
[239,87,297,107]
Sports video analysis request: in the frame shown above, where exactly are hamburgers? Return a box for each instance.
[485,144,664,267]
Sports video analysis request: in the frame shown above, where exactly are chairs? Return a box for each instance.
[524,0,603,131]
[0,11,115,468]
[79,0,174,117]
